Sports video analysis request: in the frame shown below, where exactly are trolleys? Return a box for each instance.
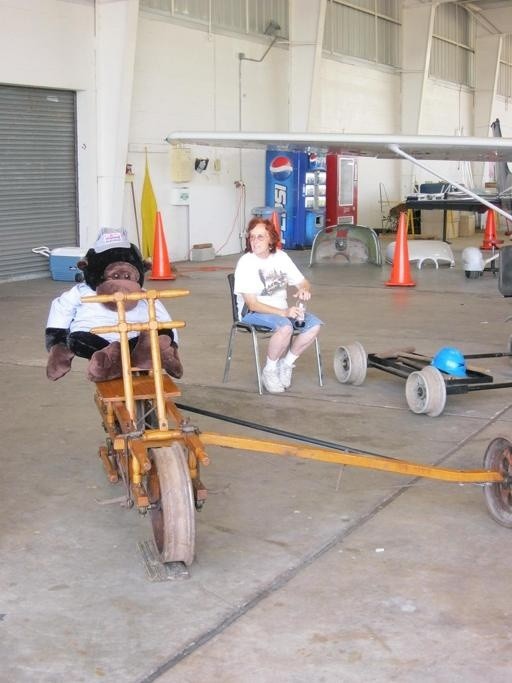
[334,341,511,415]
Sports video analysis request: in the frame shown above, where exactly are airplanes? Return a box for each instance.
[164,131,511,299]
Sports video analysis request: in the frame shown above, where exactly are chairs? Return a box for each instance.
[225,271,325,395]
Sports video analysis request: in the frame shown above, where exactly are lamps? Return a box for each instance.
[237,19,290,61]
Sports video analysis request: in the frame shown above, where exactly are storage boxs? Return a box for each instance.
[49,245,89,281]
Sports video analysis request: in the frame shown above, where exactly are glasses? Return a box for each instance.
[249,236,265,241]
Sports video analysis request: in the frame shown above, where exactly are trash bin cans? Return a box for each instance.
[497,243,512,297]
[251,206,283,226]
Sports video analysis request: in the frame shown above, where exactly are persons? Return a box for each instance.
[234,218,324,393]
[195,155,210,173]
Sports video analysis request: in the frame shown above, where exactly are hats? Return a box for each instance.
[95,227,130,253]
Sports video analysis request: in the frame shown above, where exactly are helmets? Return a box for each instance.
[432,347,466,377]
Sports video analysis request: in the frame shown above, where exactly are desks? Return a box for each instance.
[403,195,512,246]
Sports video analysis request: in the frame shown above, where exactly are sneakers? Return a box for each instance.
[262,359,296,393]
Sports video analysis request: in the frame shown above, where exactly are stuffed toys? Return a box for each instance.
[44,227,183,382]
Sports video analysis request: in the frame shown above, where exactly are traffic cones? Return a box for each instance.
[147,211,176,280]
[479,209,500,249]
[385,212,415,287]
